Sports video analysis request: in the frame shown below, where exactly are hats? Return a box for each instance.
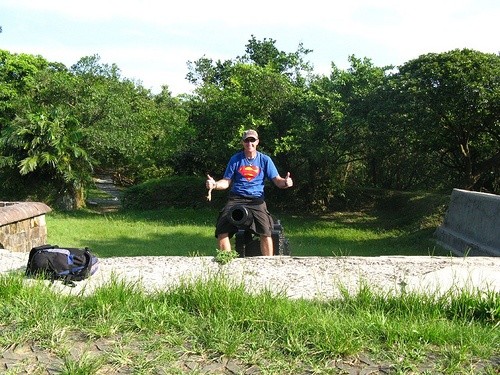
[243,129,259,141]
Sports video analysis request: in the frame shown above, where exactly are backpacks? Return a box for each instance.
[25,244,99,287]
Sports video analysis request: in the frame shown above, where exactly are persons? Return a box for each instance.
[206,129,293,256]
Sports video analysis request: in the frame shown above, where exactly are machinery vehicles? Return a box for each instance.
[228,204,285,258]
